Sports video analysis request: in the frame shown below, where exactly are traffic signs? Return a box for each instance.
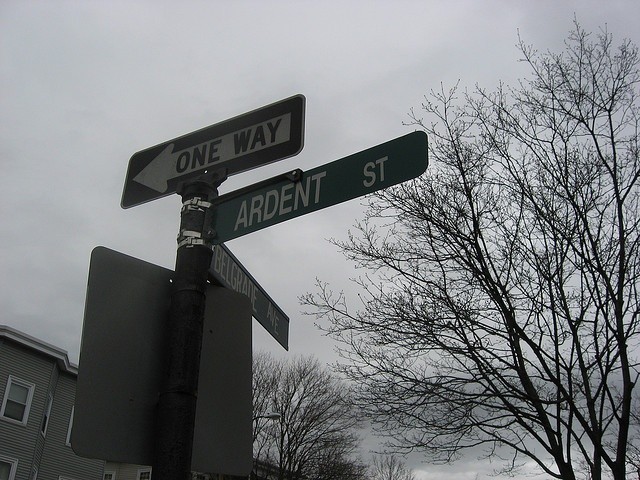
[208,243,290,351]
[121,95,305,210]
[211,131,428,245]
